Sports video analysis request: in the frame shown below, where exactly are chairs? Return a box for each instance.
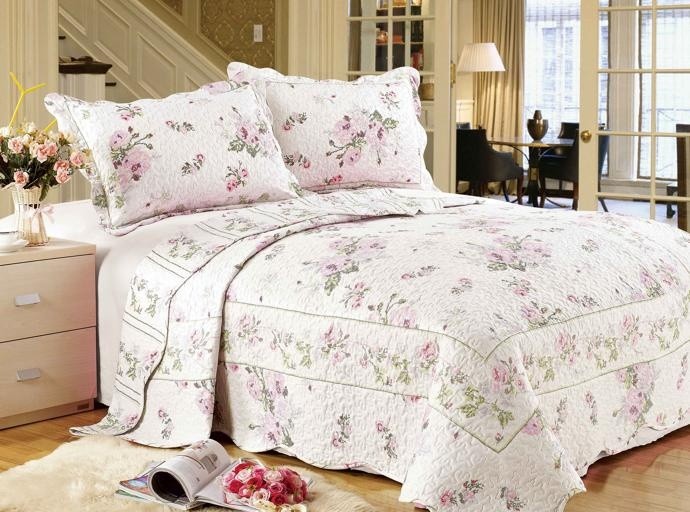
[454,127,524,202]
[539,120,612,212]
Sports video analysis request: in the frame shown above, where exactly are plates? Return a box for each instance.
[0,239,29,253]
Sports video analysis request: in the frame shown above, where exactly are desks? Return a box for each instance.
[486,137,575,209]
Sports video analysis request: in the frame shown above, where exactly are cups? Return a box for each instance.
[527,119,549,141]
[7,229,25,243]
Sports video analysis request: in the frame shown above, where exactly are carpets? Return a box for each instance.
[1,431,374,512]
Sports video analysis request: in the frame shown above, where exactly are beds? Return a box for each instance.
[5,187,688,512]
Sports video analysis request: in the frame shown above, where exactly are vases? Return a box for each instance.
[11,187,50,246]
[526,110,549,141]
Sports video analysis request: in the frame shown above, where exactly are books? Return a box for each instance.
[114,438,315,512]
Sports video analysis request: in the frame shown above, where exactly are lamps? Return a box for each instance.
[456,42,508,127]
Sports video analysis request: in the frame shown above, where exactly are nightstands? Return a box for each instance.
[0,235,101,434]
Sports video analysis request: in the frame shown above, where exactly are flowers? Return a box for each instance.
[217,459,312,512]
[0,117,95,201]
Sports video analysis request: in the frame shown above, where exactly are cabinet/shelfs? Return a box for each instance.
[346,0,440,99]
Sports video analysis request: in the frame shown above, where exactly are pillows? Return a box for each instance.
[226,61,433,191]
[44,81,301,235]
